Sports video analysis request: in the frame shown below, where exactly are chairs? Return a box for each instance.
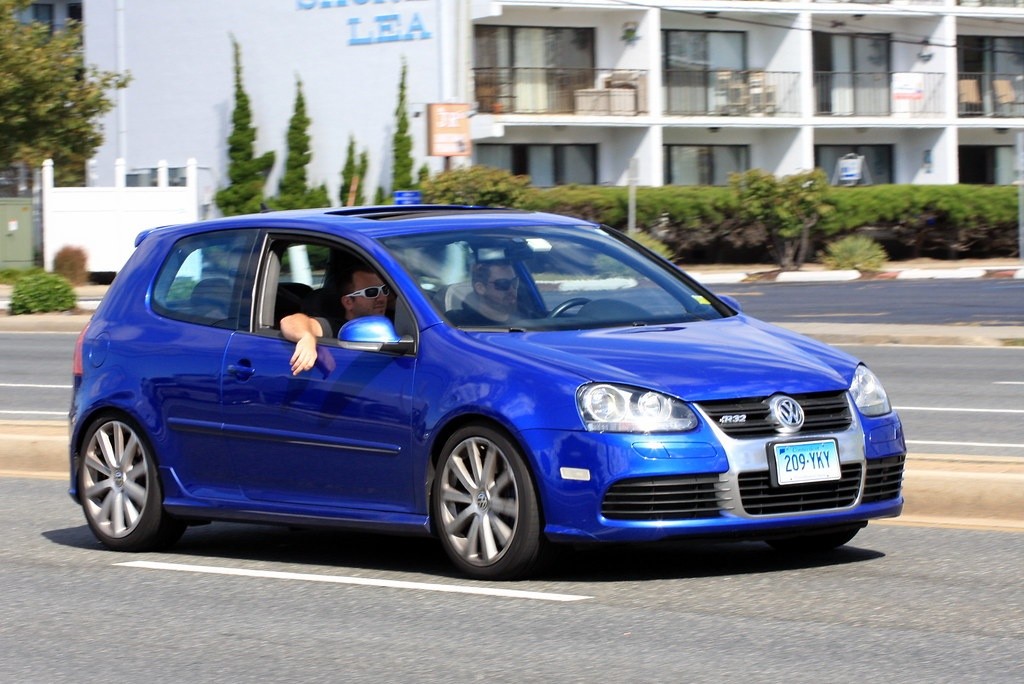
[300,290,344,322]
[958,79,982,116]
[993,80,1024,118]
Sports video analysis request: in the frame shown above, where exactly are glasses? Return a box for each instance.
[488,275,520,292]
[343,284,390,299]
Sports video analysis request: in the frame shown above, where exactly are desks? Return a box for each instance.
[573,89,636,116]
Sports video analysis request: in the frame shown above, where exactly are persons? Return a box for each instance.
[444,259,520,327]
[279,264,390,376]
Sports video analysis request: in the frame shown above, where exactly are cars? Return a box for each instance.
[69,206,908,578]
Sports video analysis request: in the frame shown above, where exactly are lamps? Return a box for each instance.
[994,128,1009,135]
[853,15,866,20]
